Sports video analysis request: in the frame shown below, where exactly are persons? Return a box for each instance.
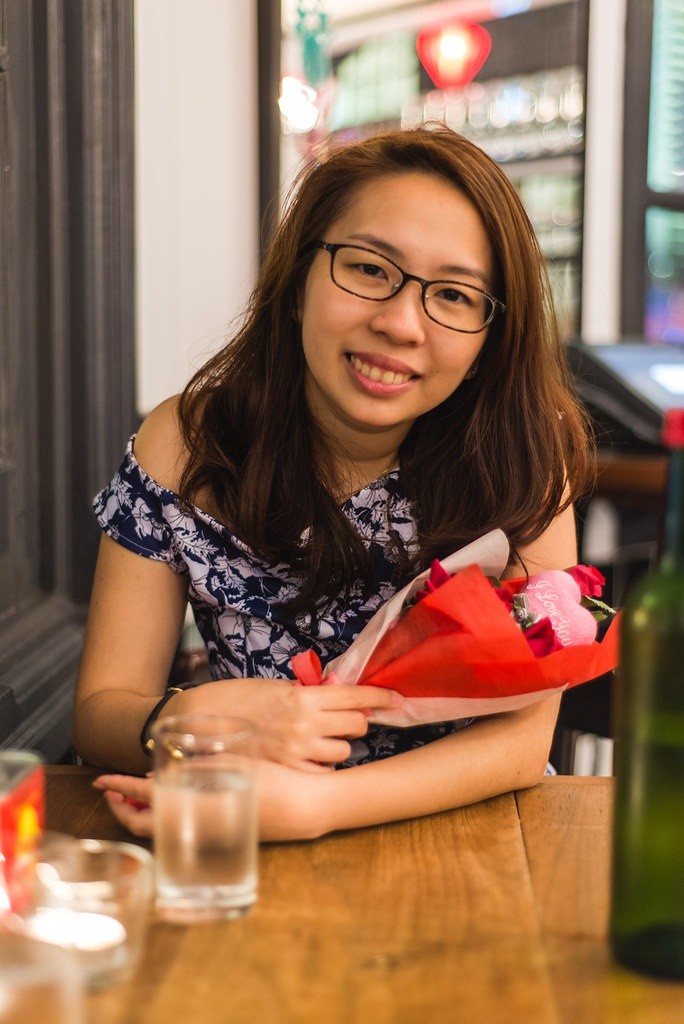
[73,118,595,842]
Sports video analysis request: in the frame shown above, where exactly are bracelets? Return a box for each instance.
[139,688,179,761]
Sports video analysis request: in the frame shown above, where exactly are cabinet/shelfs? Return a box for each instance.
[419,0,591,344]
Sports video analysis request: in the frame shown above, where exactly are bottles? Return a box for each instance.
[610,407,684,981]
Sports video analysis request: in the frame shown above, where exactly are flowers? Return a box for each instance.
[403,559,615,658]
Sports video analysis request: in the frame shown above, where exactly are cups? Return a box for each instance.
[155,717,255,915]
[0,840,156,1024]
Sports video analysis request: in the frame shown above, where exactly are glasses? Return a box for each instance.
[315,239,507,334]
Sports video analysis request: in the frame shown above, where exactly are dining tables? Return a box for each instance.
[0,764,684,1024]
